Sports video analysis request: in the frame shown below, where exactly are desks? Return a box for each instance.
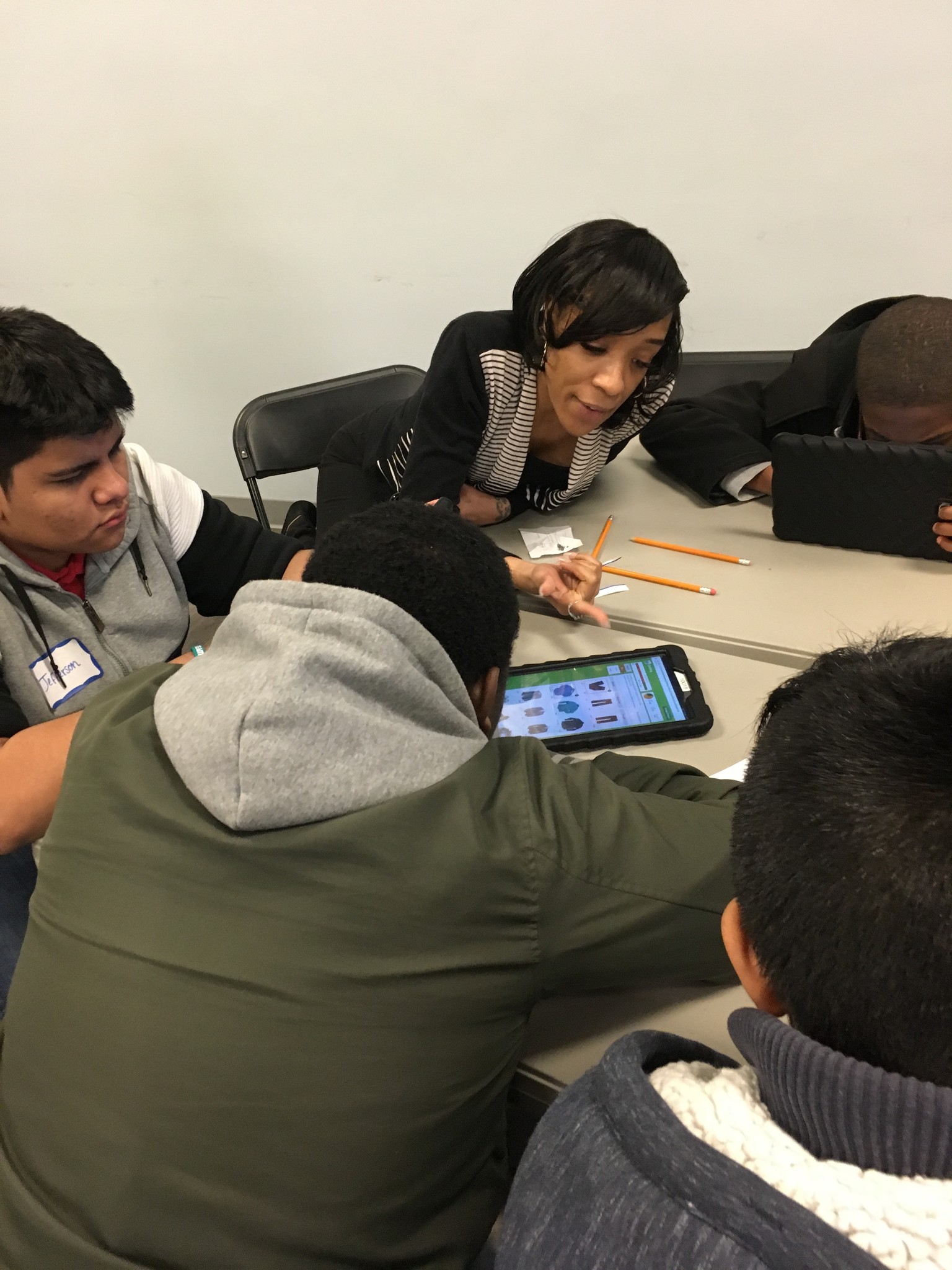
[475,422,951,1108]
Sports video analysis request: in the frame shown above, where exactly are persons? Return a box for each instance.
[0,499,750,1270]
[482,615,952,1270]
[639,294,952,551]
[0,307,314,1020]
[279,217,690,537]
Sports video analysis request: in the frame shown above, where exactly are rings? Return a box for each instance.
[568,600,584,621]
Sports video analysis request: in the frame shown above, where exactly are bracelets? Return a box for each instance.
[189,644,204,661]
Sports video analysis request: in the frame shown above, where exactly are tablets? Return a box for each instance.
[490,646,713,754]
[771,433,952,563]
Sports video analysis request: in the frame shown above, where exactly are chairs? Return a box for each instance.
[232,348,799,529]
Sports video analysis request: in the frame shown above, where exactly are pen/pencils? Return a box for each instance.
[602,566,717,595]
[592,515,614,558]
[629,537,751,566]
